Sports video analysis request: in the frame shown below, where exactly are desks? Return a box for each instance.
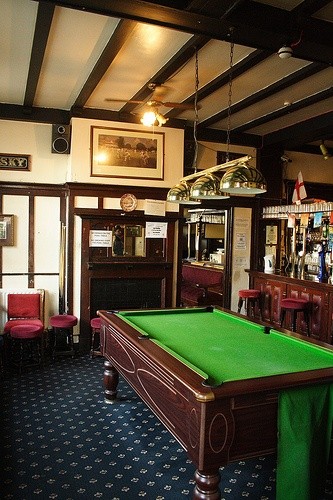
[96,304,333,500]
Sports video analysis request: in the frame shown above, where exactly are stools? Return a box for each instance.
[238,290,261,317]
[279,298,311,336]
[10,325,42,373]
[89,318,103,359]
[49,316,77,360]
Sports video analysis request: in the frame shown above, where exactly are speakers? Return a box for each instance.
[51,124,71,154]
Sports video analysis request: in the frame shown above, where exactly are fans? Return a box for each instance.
[104,82,200,112]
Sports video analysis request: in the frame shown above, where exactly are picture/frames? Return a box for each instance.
[0,213,14,246]
[89,125,166,182]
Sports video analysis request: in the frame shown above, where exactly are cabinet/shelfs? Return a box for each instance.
[244,201,333,346]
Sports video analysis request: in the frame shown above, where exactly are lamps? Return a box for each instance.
[141,108,169,128]
[167,24,267,206]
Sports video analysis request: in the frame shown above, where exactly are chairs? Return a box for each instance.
[3,294,43,363]
